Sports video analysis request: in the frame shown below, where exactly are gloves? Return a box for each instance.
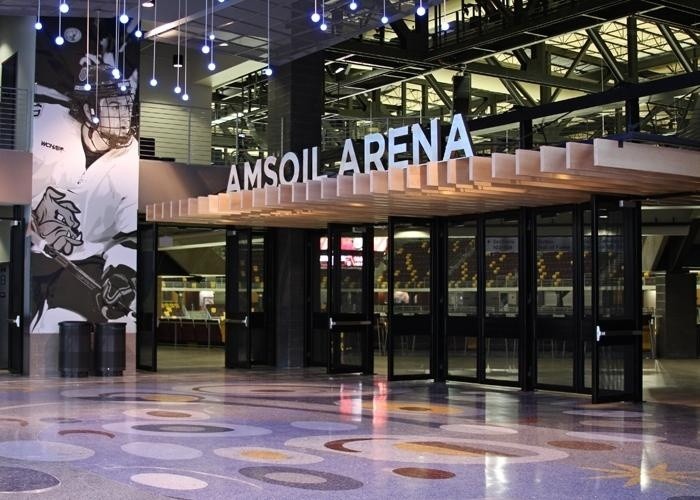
[97,264,137,320]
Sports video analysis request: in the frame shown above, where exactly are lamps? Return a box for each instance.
[34,0,231,127]
[264,0,275,80]
[312,1,450,33]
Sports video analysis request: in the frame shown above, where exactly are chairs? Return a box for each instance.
[370,312,655,360]
[158,316,221,349]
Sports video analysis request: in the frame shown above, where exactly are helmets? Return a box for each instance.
[70,62,134,147]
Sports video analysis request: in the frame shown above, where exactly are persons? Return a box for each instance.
[26,63,138,336]
[390,281,410,304]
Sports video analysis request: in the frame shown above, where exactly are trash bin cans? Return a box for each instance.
[59,321,127,377]
